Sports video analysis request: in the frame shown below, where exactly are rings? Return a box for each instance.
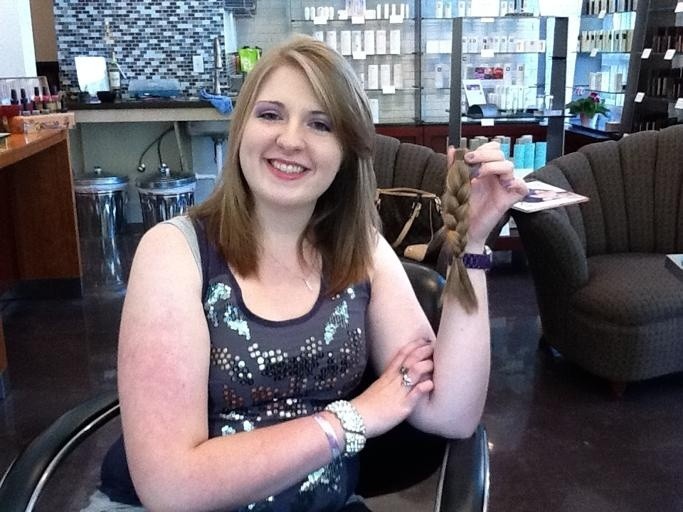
[402,375,412,387]
[399,366,409,374]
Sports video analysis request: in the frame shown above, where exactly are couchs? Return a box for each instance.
[370,131,477,264]
[514,123,683,389]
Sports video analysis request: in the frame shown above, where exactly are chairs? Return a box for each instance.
[1,261,489,512]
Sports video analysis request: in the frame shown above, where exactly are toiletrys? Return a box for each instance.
[10,86,59,111]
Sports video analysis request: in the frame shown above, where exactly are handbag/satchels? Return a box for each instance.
[374,187,443,247]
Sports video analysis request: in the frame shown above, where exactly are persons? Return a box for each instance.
[81,39,531,512]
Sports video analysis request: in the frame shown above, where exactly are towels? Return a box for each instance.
[202,90,232,116]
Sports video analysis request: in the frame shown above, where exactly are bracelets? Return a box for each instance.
[312,411,340,461]
[324,398,367,457]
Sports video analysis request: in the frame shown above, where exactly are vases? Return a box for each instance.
[579,112,598,123]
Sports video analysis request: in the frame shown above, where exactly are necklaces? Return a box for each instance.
[265,249,318,293]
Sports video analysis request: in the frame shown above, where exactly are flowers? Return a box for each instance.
[564,91,612,118]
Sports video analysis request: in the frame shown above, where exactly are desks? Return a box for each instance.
[0,131,83,304]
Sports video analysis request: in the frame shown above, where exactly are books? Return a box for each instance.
[512,178,590,214]
[632,35,683,131]
[665,254,683,279]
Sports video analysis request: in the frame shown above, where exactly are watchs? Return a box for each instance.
[463,244,495,269]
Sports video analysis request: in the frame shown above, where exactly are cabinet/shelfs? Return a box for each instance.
[291,4,415,122]
[376,125,479,153]
[419,2,581,119]
[571,4,652,130]
[632,2,683,135]
